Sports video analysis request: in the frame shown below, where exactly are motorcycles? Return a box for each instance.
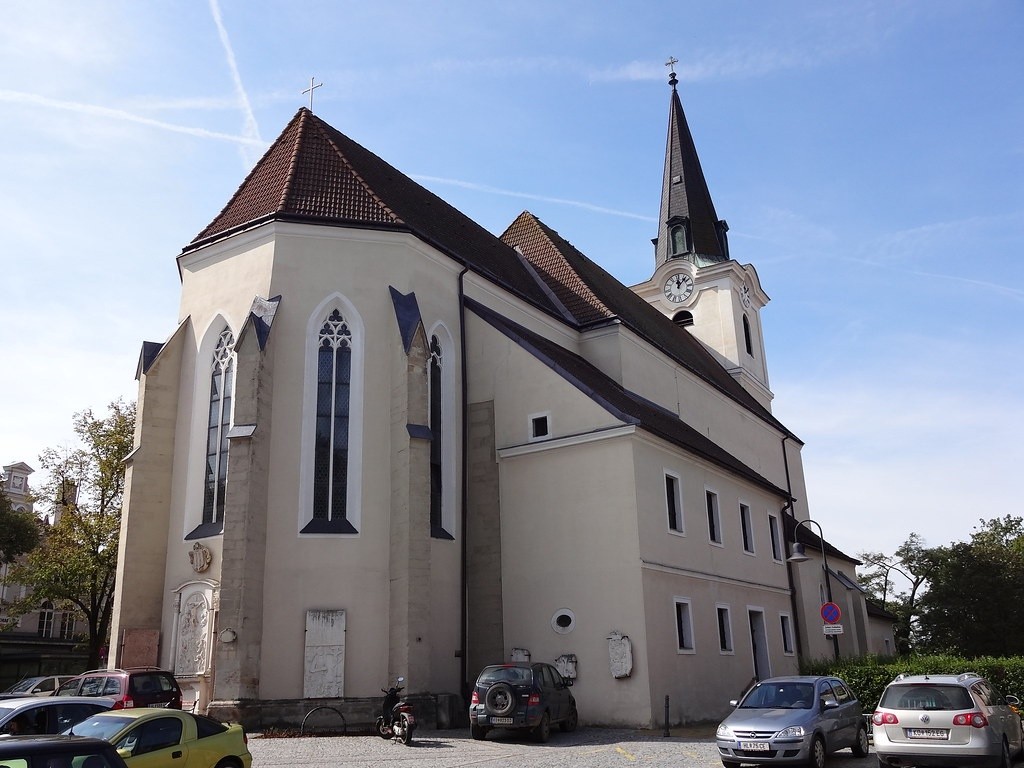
[376,676,418,746]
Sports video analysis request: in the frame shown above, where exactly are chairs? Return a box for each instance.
[32,712,46,733]
[82,756,104,768]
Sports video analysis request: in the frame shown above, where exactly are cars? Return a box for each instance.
[715,674,871,768]
[0,674,81,702]
[0,707,254,768]
[0,695,123,736]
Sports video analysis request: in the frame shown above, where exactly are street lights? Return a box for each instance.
[786,518,841,664]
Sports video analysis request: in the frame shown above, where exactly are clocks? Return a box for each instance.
[660,269,699,311]
[739,280,752,311]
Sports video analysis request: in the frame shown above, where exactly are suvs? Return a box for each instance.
[872,670,1024,768]
[49,664,182,711]
[1,732,129,768]
[468,661,579,743]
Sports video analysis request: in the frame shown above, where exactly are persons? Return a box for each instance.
[185,601,205,623]
